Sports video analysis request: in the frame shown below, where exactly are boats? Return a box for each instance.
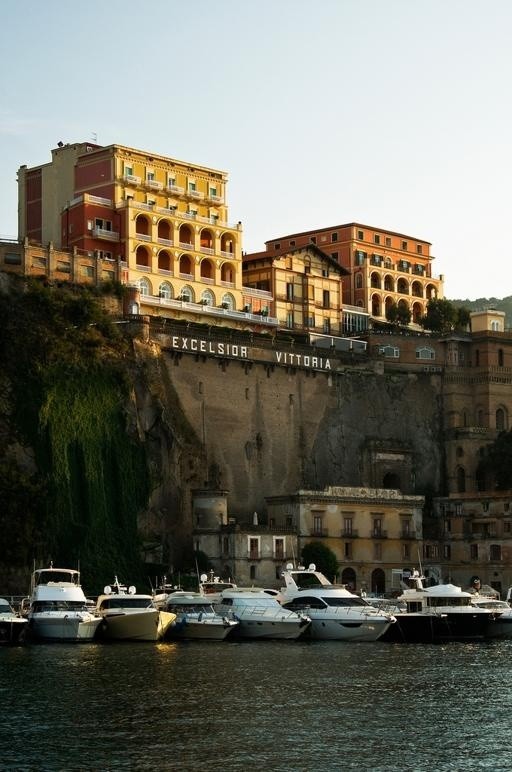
[146,572,240,644]
[393,548,503,638]
[192,558,313,642]
[468,592,512,635]
[93,573,179,645]
[0,597,30,644]
[268,535,399,645]
[18,557,105,644]
